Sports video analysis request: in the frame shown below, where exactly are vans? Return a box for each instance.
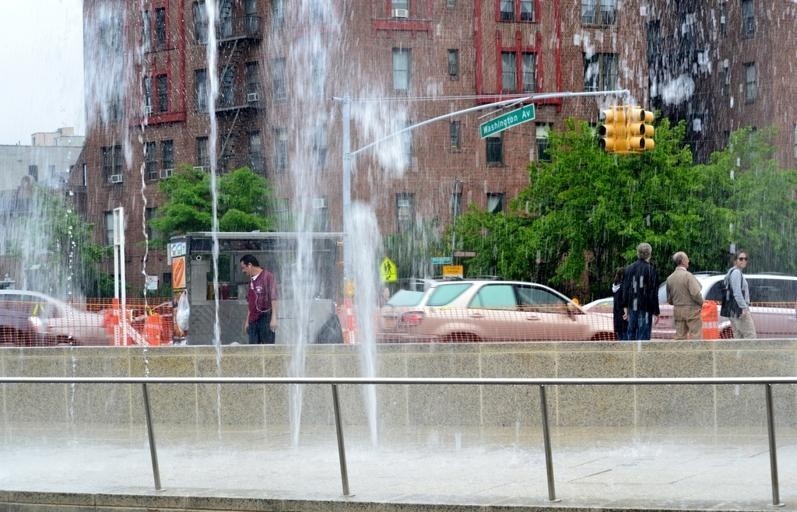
[654,273,797,337]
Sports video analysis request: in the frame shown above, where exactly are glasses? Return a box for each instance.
[739,257,747,261]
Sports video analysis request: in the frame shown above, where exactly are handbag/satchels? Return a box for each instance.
[720,269,745,318]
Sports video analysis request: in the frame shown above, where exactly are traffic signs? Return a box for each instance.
[480,102,536,140]
[378,256,398,283]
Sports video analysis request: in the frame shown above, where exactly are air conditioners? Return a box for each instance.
[246,93,259,101]
[192,166,205,175]
[110,175,122,183]
[159,169,173,179]
[391,9,408,18]
[310,196,324,207]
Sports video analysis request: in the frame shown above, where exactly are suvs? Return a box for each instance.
[376,279,614,342]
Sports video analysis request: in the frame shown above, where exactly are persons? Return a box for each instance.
[611,262,636,340]
[725,250,756,340]
[636,231,658,338]
[238,254,276,346]
[666,251,704,338]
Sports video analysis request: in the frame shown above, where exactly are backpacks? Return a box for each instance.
[704,267,744,319]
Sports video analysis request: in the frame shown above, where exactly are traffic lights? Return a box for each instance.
[596,106,656,155]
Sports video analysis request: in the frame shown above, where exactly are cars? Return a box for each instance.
[132,303,185,337]
[0,289,114,346]
